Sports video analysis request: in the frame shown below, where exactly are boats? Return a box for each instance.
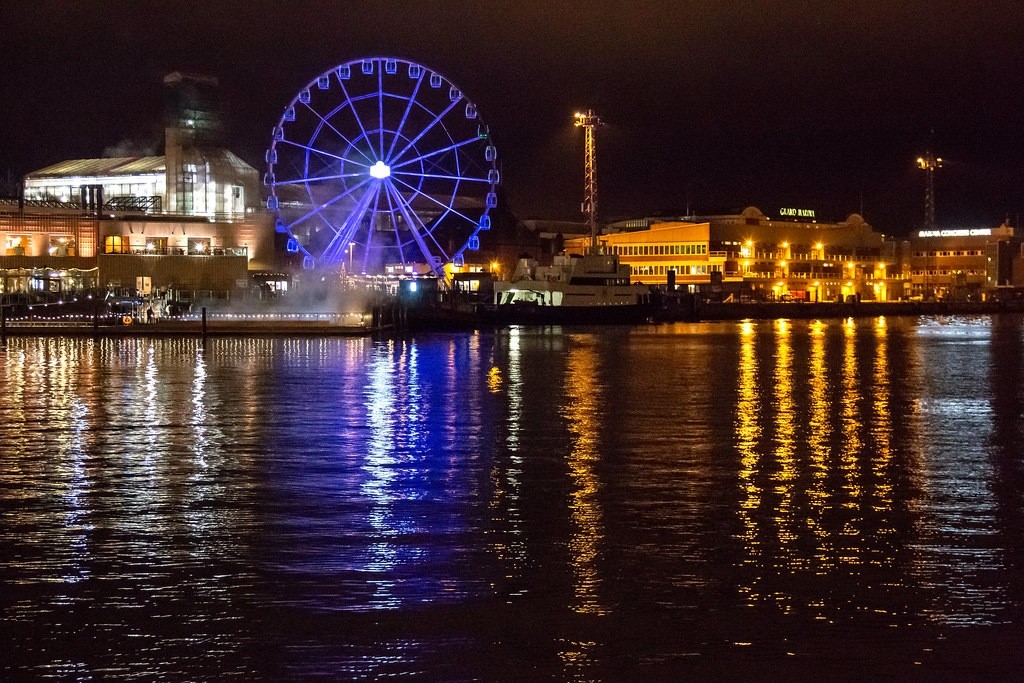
[484,253,655,322]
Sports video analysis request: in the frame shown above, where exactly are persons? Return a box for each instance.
[146,306,153,324]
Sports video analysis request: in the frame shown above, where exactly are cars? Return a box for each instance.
[780,295,801,302]
